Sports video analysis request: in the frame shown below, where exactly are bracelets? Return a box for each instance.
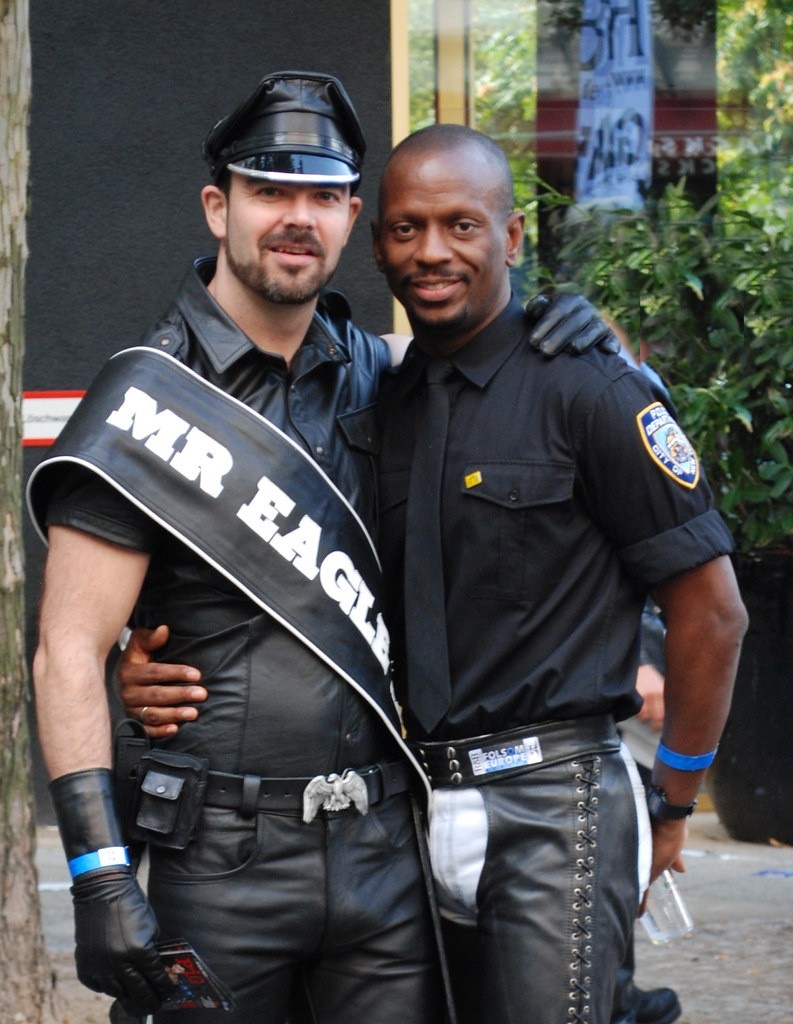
[68,846,130,880]
[655,740,718,771]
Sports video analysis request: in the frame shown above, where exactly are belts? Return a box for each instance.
[416,711,622,789]
[137,752,413,819]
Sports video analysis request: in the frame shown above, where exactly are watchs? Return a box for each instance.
[646,783,697,821]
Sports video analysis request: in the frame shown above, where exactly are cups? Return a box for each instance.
[641,868,694,947]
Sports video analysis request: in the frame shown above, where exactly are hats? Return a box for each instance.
[200,69,367,186]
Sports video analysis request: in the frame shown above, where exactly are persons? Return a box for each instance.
[164,963,184,985]
[113,124,750,1024]
[26,70,621,1023]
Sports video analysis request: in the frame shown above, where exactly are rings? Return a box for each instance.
[140,707,148,720]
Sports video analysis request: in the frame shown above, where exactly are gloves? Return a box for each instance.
[526,288,622,359]
[46,766,182,1017]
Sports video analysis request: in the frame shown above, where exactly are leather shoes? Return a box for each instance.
[611,988,682,1024]
